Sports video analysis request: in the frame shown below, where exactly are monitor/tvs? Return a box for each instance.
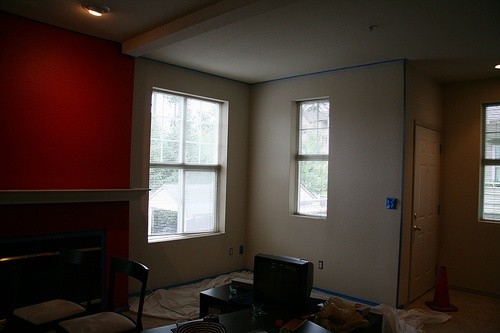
[255,253,314,311]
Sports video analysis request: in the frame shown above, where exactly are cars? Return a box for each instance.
[300,184,327,215]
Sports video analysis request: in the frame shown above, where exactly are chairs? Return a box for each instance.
[58,255,150,333]
[12,250,95,333]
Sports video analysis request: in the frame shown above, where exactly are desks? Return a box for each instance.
[141,297,384,333]
[199,280,254,321]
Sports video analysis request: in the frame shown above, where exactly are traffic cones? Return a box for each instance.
[425,266,459,312]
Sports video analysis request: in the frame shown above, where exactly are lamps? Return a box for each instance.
[81,2,112,16]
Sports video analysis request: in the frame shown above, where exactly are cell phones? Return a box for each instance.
[283,318,306,331]
